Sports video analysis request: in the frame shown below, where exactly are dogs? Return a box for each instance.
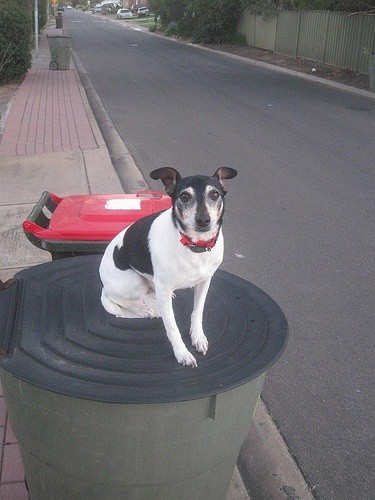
[98,167,237,368]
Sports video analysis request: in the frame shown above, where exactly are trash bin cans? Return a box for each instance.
[47,34,74,71]
[21,189,173,261]
[1,254,291,500]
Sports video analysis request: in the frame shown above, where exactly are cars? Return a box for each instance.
[116,9,132,19]
[66,5,72,10]
[131,5,150,17]
[92,2,115,15]
